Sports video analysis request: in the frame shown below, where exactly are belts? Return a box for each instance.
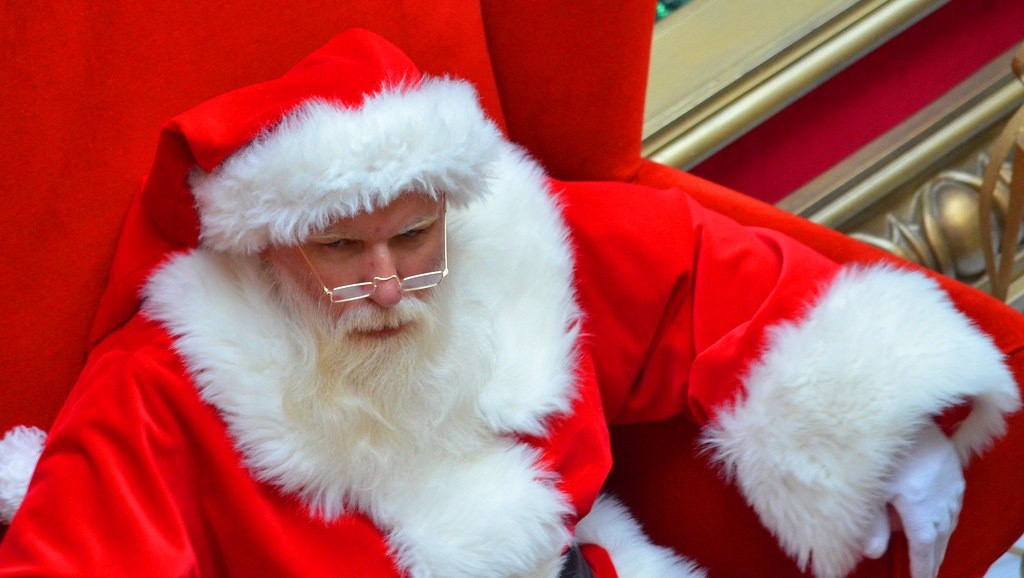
[559,544,593,578]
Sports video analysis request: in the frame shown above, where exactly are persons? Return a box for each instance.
[0,30,1018,578]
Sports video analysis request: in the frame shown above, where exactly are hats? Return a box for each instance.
[0,28,494,529]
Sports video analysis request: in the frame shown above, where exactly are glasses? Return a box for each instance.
[294,193,449,304]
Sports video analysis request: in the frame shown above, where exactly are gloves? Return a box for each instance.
[861,416,964,578]
